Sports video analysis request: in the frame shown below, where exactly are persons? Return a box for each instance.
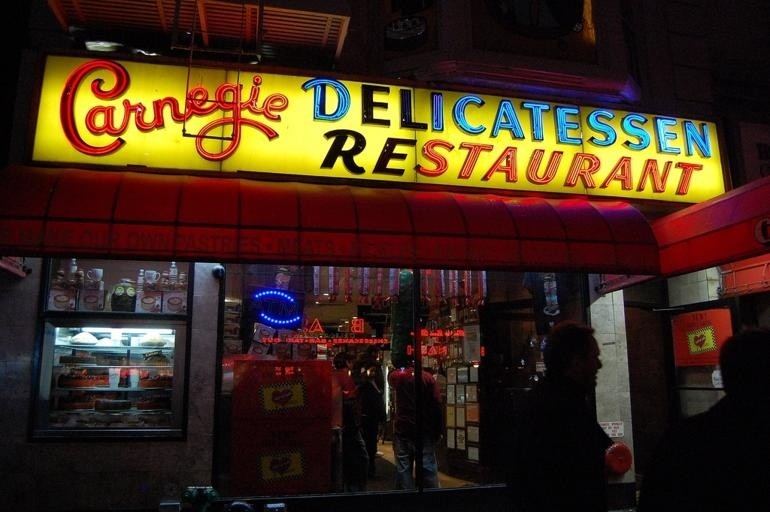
[387,354,444,490]
[333,345,386,491]
[639,330,770,511]
[519,321,631,512]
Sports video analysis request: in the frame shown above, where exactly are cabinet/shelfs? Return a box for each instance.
[33,311,187,437]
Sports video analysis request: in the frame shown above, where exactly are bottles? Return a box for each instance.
[68,258,85,289]
[162,261,187,284]
[136,269,144,291]
[56,271,64,283]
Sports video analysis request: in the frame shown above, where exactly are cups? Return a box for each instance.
[87,269,104,281]
[145,270,160,283]
